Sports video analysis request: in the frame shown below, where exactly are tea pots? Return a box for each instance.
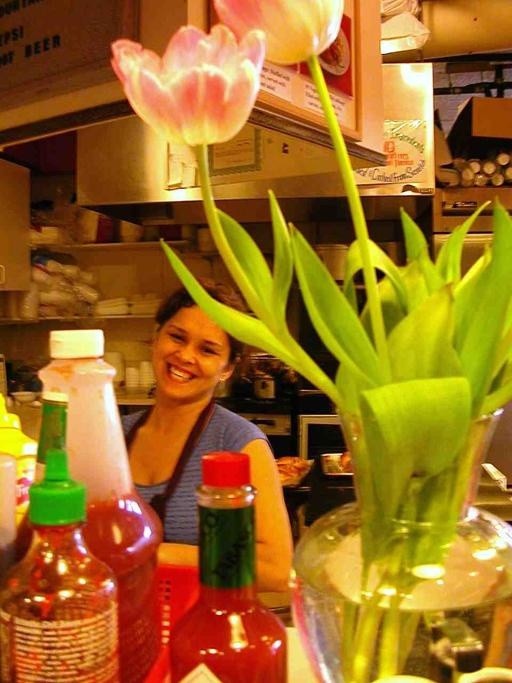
[243,368,276,400]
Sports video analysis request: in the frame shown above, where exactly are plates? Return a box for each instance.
[319,27,351,76]
[97,296,163,315]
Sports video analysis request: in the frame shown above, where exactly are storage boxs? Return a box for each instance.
[431,233,493,279]
[445,95,512,160]
[423,184,512,234]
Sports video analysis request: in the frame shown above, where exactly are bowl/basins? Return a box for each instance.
[117,218,217,253]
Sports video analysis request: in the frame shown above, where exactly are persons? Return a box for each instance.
[121,284,294,593]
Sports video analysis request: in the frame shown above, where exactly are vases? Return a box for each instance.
[290,499,512,683]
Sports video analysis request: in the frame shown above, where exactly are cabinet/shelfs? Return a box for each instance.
[0,232,193,407]
[0,158,35,293]
[227,402,298,458]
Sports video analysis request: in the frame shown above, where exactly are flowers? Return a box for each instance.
[108,0,512,682]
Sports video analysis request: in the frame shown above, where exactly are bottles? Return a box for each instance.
[0,449,120,682]
[166,452,289,682]
[13,329,165,681]
[2,392,38,547]
